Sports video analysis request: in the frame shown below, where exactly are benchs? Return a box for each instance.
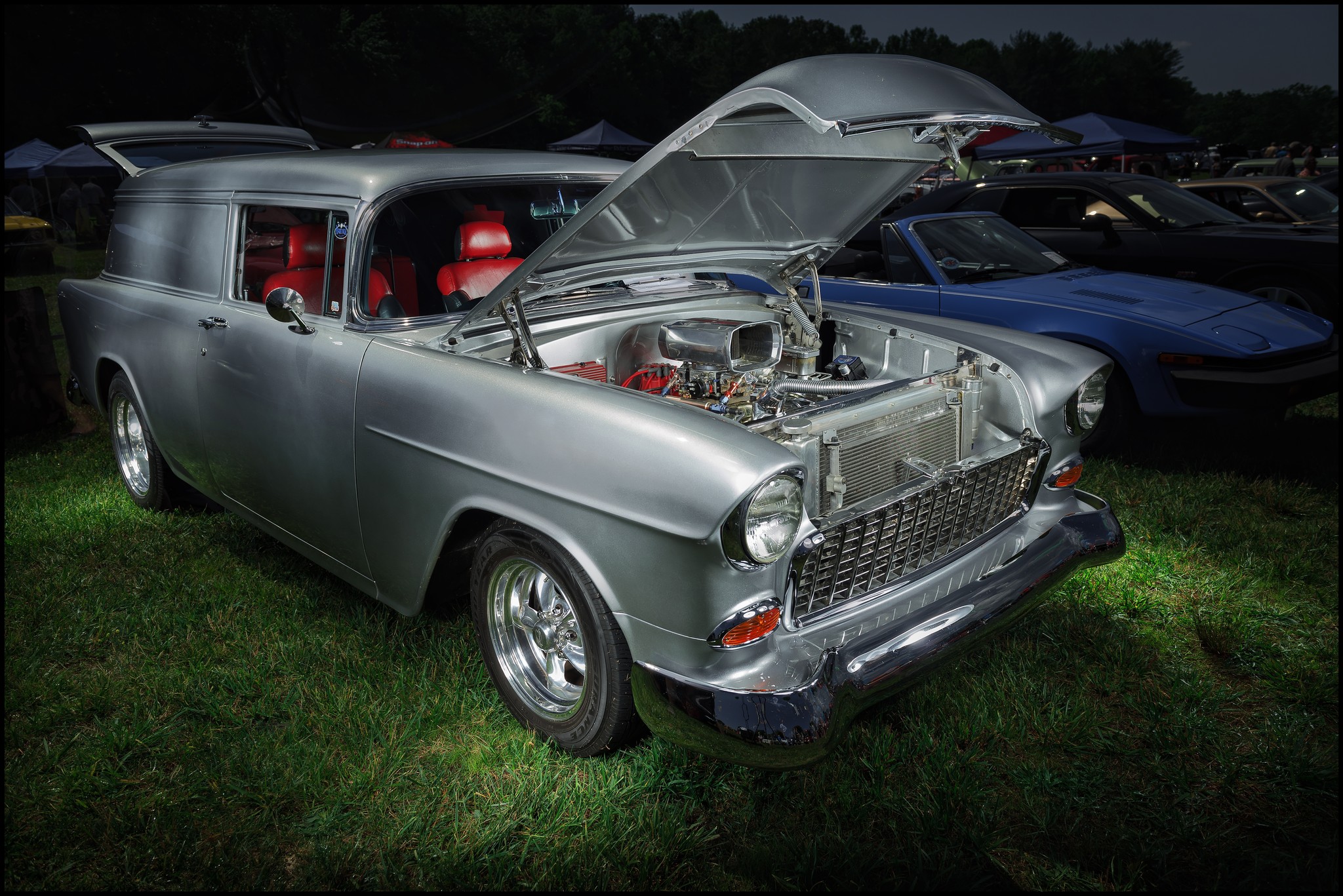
[248,252,421,319]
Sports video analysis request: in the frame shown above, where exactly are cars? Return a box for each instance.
[872,147,1342,227]
[56,47,1127,753]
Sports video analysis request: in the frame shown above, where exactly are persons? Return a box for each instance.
[1132,150,1220,177]
[1264,142,1325,178]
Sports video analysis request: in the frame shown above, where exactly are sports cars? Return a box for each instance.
[707,209,1343,459]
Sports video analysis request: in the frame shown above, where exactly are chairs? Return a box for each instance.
[263,221,409,321]
[436,220,527,310]
[851,250,888,281]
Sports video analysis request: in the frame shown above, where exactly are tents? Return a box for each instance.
[3,135,171,245]
[965,111,1201,173]
